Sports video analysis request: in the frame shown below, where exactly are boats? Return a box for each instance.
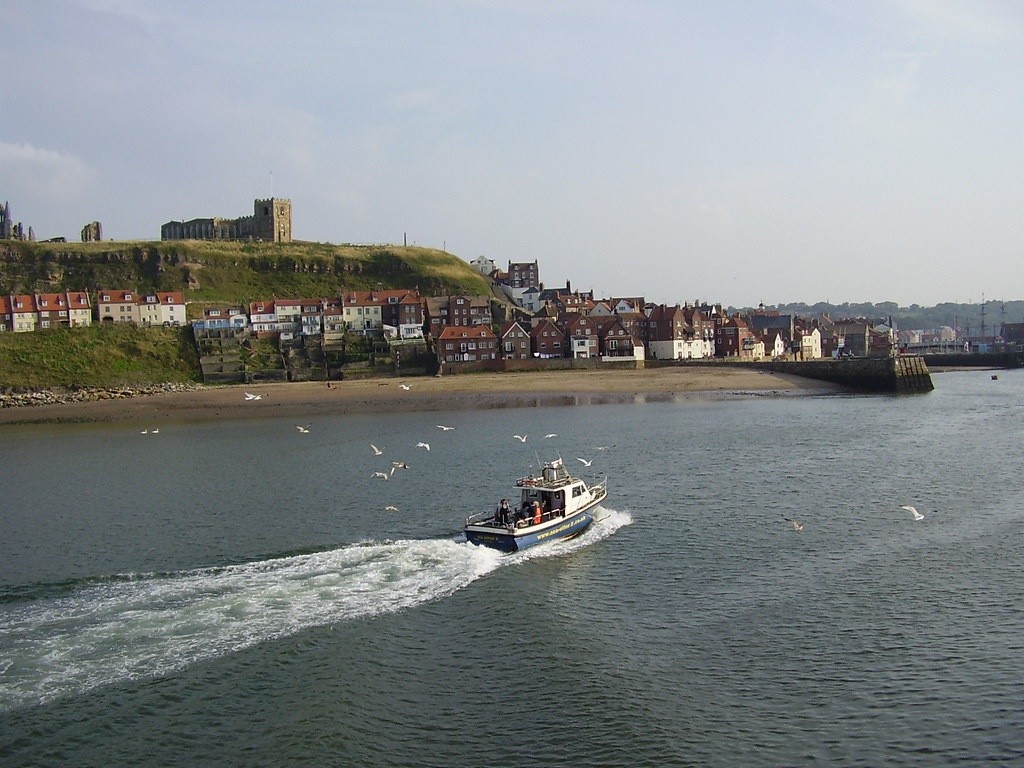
[464,453,609,554]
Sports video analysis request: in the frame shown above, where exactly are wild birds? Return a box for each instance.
[576,457,594,467]
[384,505,398,512]
[415,442,430,451]
[595,444,616,452]
[436,425,457,431]
[244,392,262,401]
[296,422,311,433]
[370,444,386,457]
[140,428,159,434]
[541,434,560,440]
[790,519,804,531]
[514,435,528,443]
[369,461,409,480]
[896,504,924,521]
[398,384,412,391]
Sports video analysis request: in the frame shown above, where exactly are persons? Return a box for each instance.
[495,497,542,526]
